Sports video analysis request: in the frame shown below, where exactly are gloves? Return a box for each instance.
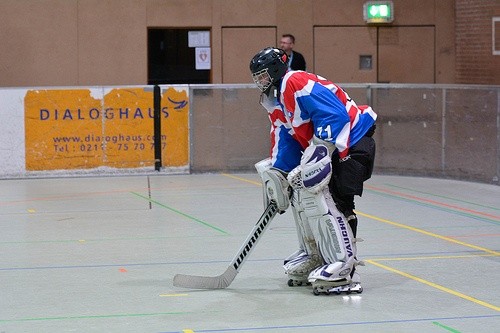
[256,157,293,214]
[286,143,333,196]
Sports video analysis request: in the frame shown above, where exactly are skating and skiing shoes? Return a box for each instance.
[284,269,313,287]
[312,264,364,296]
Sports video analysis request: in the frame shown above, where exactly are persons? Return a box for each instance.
[250,47,378,295]
[280,34,308,71]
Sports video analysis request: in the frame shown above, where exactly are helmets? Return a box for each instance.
[249,47,289,99]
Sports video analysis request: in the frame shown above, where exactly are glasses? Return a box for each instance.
[280,41,291,45]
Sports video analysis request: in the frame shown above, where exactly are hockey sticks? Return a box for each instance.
[174,183,295,289]
